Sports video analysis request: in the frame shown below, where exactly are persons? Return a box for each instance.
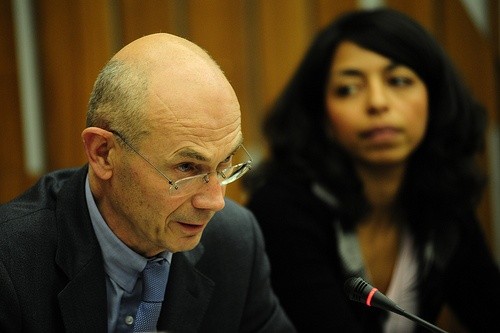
[0,32,296,333]
[241,9,500,333]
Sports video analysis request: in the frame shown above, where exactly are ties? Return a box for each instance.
[132,259,170,333]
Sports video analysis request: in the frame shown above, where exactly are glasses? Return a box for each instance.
[105,129,254,195]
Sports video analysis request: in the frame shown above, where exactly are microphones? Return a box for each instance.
[344,276,448,333]
